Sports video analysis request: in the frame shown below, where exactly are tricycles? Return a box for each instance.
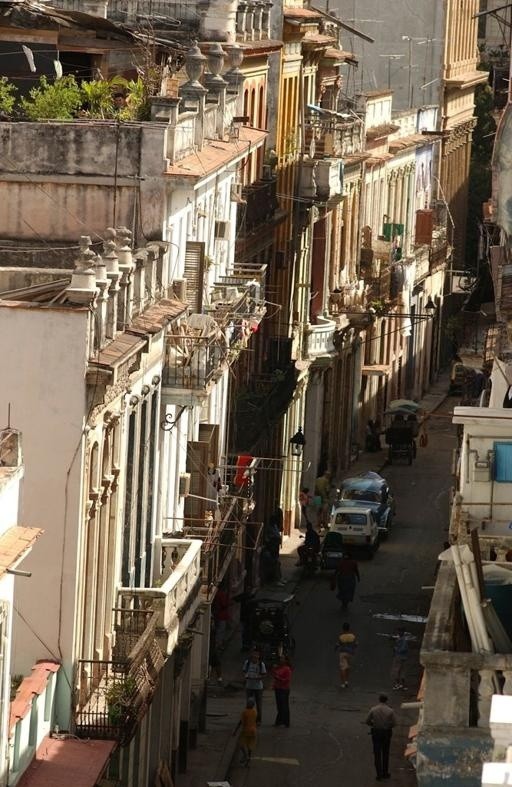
[243,594,297,673]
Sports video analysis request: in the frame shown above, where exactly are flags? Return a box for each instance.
[233,454,254,486]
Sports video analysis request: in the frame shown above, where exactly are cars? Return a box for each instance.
[331,475,396,541]
[326,505,379,560]
[449,362,476,395]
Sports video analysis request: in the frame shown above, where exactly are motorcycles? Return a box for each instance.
[299,528,348,576]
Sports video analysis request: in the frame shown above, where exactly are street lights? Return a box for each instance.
[265,423,308,580]
[405,290,438,405]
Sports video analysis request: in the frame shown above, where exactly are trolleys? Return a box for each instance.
[385,399,419,464]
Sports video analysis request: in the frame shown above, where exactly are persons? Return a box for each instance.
[210,582,234,650]
[240,649,268,726]
[209,617,224,683]
[231,699,259,768]
[335,552,361,609]
[333,621,361,688]
[299,469,339,527]
[270,652,296,728]
[294,521,321,568]
[239,580,258,651]
[272,610,286,661]
[365,419,374,450]
[373,420,382,450]
[366,693,397,781]
[391,626,410,692]
